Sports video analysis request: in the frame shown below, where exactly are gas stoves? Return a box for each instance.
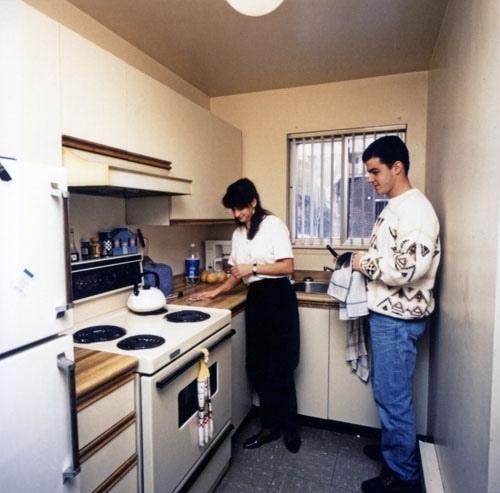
[72,304,231,375]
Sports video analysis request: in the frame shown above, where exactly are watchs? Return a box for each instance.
[252,263,257,275]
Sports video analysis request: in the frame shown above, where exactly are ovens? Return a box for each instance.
[141,323,232,493]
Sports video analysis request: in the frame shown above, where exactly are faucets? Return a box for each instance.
[323,266,333,273]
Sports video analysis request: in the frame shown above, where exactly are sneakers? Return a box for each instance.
[363,444,386,463]
[361,466,421,493]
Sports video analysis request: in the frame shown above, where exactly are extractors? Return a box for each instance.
[63,147,192,197]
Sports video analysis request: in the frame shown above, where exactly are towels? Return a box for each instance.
[196,346,217,447]
[344,318,372,384]
[325,251,370,322]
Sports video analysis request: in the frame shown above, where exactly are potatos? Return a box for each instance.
[200,271,226,284]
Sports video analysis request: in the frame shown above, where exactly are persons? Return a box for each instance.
[333,136,441,493]
[190,179,301,454]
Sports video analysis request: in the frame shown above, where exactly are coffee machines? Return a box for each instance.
[203,241,234,273]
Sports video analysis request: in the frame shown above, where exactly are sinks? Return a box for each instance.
[291,281,329,294]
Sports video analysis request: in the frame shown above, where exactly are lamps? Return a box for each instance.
[226,0,284,18]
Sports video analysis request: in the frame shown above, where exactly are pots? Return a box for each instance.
[326,244,353,270]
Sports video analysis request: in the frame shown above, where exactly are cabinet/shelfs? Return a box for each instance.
[231,310,250,437]
[58,23,172,161]
[124,63,243,226]
[75,372,141,493]
[295,305,428,437]
[0,0,62,165]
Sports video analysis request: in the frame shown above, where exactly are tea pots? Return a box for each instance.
[127,271,166,313]
[99,231,114,256]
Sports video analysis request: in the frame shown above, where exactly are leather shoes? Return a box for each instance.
[283,425,301,453]
[244,429,281,449]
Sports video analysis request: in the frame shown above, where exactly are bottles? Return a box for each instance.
[80,239,91,259]
[70,228,79,262]
[92,238,100,258]
[225,267,232,280]
[185,243,200,285]
[208,265,215,273]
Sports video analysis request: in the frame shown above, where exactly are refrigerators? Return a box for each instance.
[0,142,81,493]
